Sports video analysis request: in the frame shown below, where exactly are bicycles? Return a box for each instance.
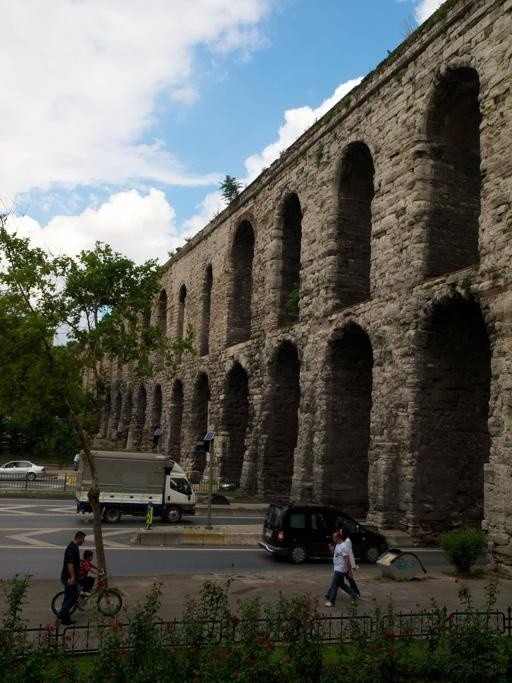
[51,569,123,618]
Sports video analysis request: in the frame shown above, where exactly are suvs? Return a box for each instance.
[257,499,388,566]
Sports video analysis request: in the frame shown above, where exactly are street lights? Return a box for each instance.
[154,428,163,453]
[202,430,215,529]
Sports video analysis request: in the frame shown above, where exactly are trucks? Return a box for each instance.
[74,447,197,525]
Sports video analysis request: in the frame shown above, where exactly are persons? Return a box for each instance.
[323,531,360,607]
[325,526,360,600]
[56,530,86,626]
[77,549,106,598]
[72,453,79,472]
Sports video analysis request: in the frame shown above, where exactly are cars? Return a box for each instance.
[0,460,48,481]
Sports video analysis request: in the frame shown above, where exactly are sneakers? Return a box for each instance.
[57,613,77,625]
[351,594,361,605]
[324,594,336,607]
